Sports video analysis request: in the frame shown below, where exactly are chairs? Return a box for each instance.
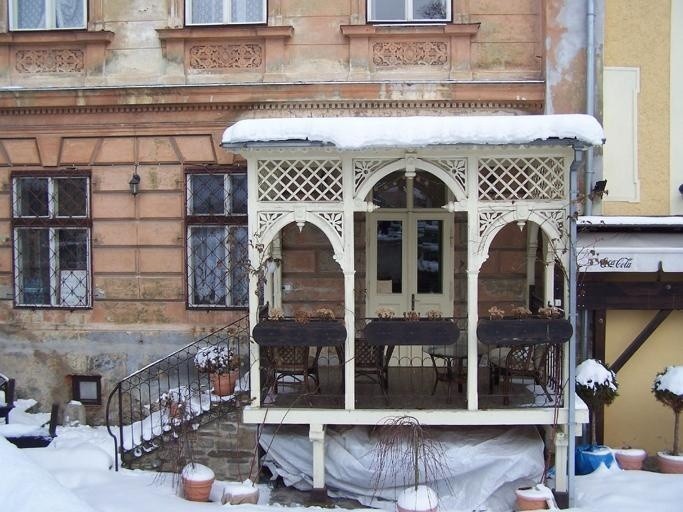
[335,338,395,405]
[263,341,323,407]
[488,339,558,404]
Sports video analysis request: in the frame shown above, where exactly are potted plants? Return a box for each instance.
[194,346,240,396]
[575,359,619,474]
[650,365,683,473]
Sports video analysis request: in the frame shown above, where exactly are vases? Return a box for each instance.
[617,453,646,470]
[169,402,186,418]
[181,477,215,501]
[514,487,548,512]
[396,504,438,512]
[223,488,259,505]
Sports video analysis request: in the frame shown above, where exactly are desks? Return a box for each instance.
[423,343,496,404]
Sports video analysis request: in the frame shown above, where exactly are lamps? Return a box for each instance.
[128,174,141,195]
